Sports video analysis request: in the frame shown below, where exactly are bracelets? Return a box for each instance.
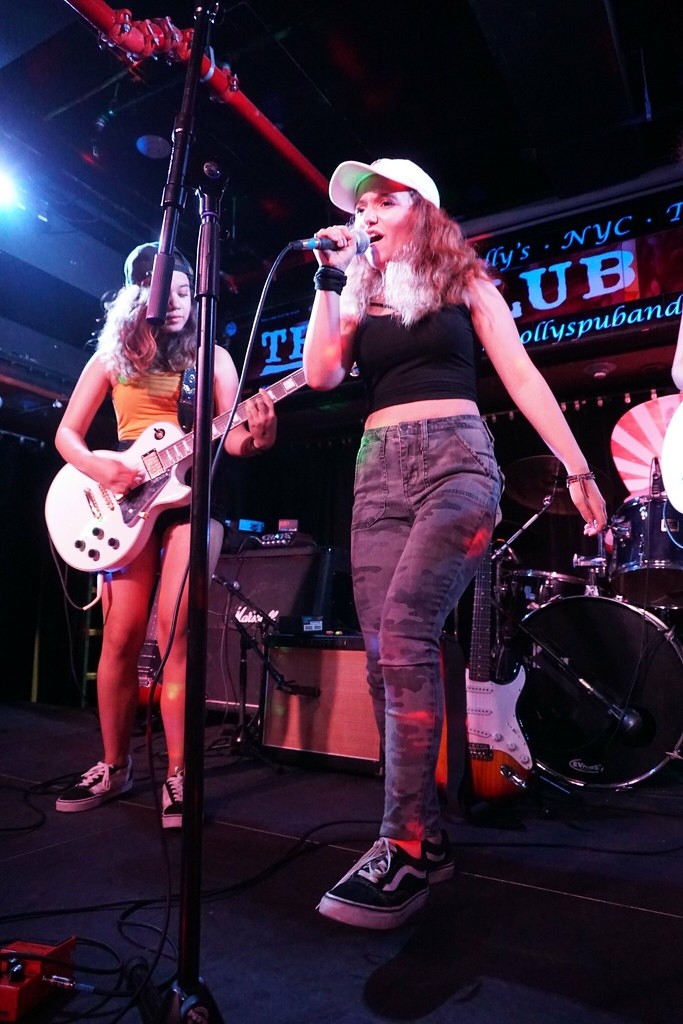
[566,472,596,486]
[250,437,262,454]
[313,265,346,295]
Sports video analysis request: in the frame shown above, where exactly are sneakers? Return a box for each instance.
[162,768,187,827]
[424,832,455,885]
[56,753,135,811]
[312,837,429,930]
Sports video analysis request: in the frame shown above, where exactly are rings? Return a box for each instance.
[314,233,318,237]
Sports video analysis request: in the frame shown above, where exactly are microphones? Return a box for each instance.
[654,458,664,492]
[621,709,643,734]
[273,684,321,698]
[291,228,371,254]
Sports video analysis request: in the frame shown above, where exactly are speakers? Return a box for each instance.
[206,545,354,718]
[255,634,387,780]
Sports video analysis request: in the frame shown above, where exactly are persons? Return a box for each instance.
[301,156,607,927]
[55,240,278,826]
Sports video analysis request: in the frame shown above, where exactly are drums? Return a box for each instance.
[492,595,683,791]
[607,494,683,611]
[493,566,605,628]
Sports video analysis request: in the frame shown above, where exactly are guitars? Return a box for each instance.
[44,366,306,575]
[432,540,534,801]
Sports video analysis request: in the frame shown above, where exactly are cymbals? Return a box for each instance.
[501,454,585,516]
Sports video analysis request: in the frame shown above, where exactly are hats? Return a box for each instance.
[124,241,195,290]
[328,158,438,216]
